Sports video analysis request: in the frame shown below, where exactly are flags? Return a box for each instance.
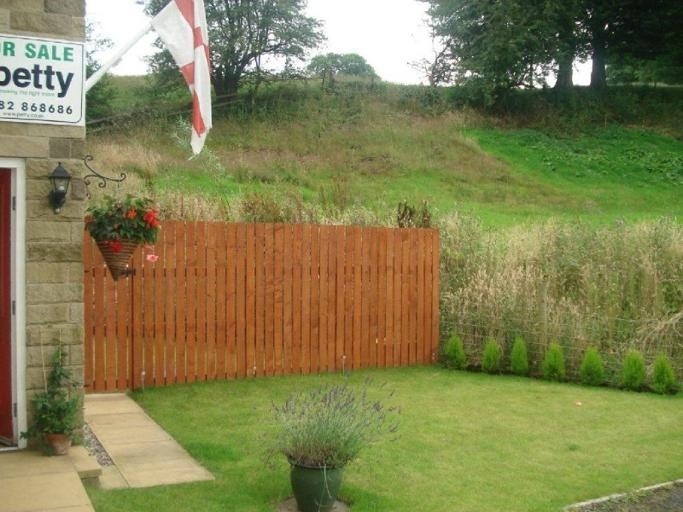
[152,0,212,161]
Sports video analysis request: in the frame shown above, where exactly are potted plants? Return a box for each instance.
[259,351,402,512]
[28,349,99,457]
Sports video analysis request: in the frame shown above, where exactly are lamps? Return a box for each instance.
[46,162,72,215]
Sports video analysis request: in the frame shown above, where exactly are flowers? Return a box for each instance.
[84,191,161,263]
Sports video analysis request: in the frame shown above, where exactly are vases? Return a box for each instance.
[93,238,139,283]
